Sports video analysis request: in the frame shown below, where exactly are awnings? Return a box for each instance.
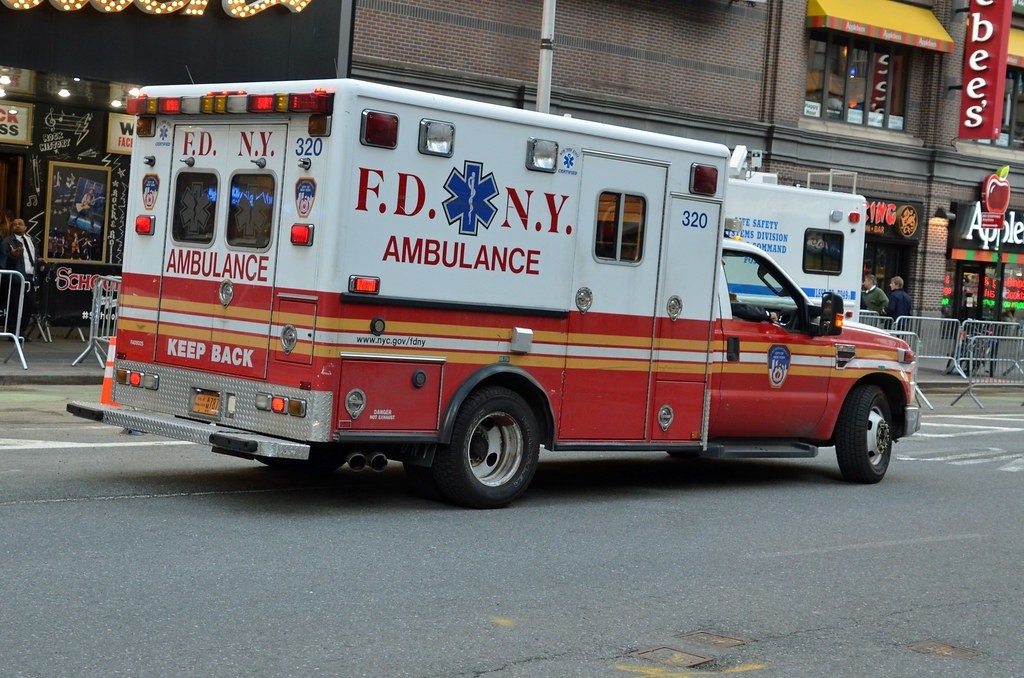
[1007,28,1024,68]
[808,0,954,54]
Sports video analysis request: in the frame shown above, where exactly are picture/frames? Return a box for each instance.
[43,161,112,264]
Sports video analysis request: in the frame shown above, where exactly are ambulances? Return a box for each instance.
[65,78,924,509]
[720,170,867,334]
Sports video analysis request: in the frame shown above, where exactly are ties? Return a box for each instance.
[21,235,35,266]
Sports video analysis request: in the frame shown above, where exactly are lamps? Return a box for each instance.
[934,207,956,220]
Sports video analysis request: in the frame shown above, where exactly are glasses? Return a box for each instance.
[13,223,24,226]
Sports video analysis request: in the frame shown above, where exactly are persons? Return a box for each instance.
[1004,301,1016,321]
[72,187,95,228]
[821,299,832,328]
[883,276,911,339]
[862,274,889,311]
[0,219,37,342]
[49,225,98,261]
[722,260,778,323]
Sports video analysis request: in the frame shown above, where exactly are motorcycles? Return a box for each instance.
[941,322,996,377]
